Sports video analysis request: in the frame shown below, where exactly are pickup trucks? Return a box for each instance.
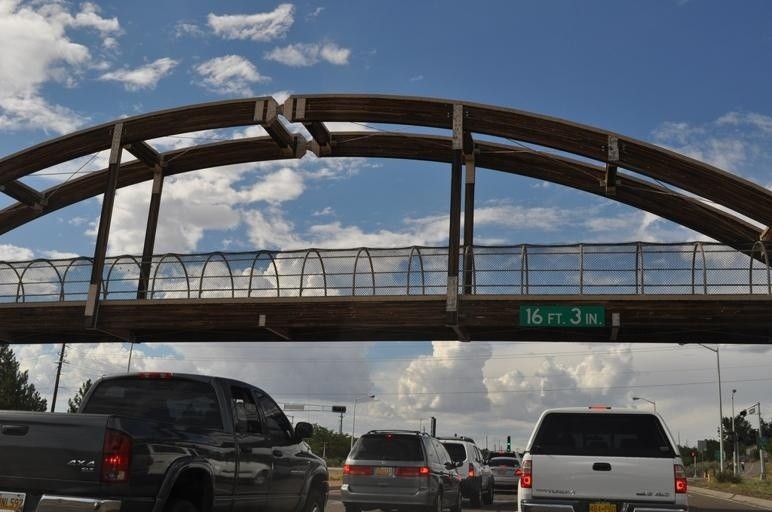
[0,372,329,512]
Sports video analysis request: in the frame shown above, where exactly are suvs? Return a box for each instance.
[341,430,521,512]
[517,406,688,511]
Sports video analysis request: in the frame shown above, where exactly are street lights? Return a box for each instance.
[732,390,737,475]
[350,396,375,452]
[634,396,656,413]
[680,342,724,473]
